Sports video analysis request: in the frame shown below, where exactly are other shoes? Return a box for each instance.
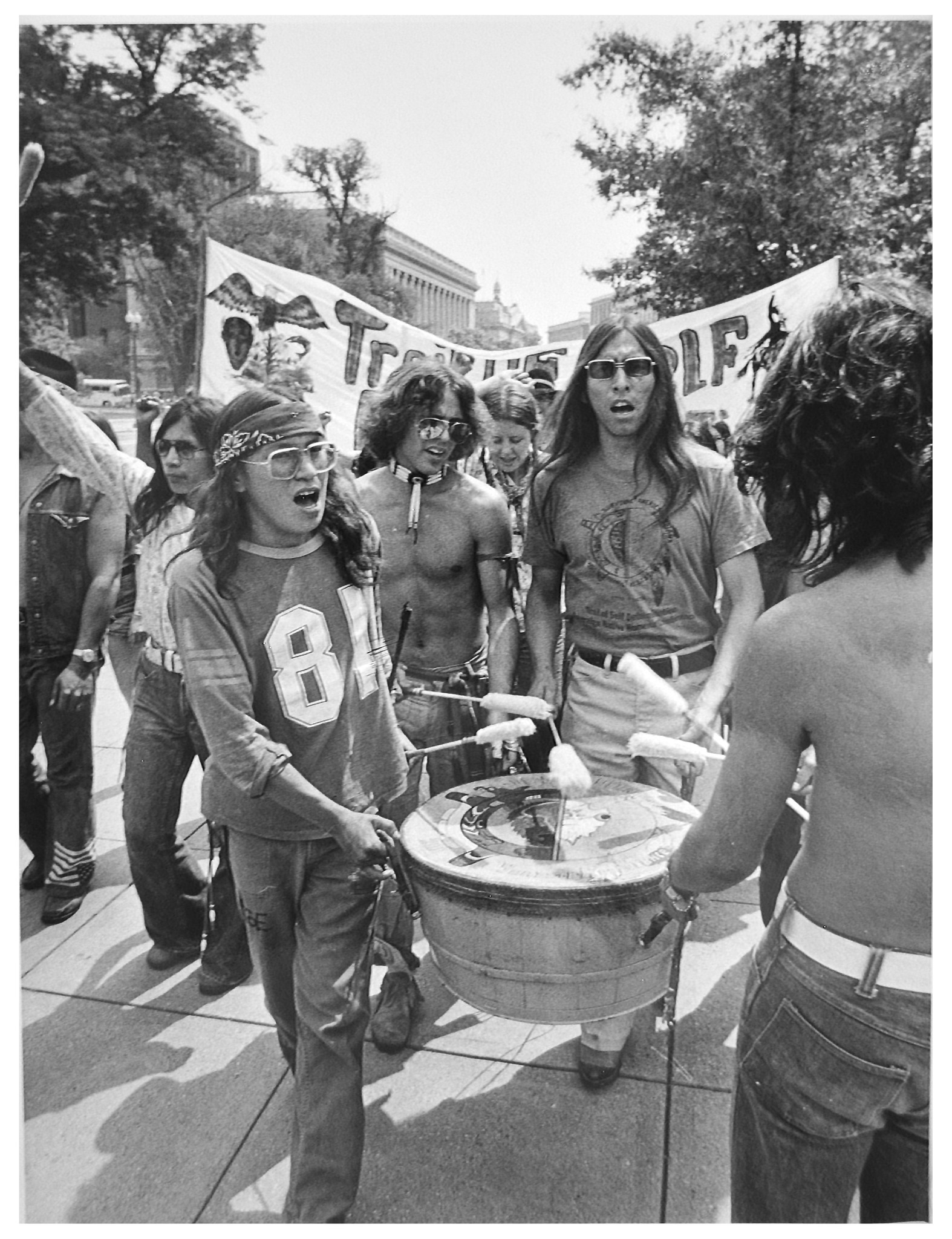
[579,1045,623,1087]
[42,886,86,924]
[199,975,233,995]
[146,943,181,970]
[22,858,47,890]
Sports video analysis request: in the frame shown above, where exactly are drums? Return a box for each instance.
[395,772,705,1027]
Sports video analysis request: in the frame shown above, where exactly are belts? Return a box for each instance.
[578,643,718,678]
[145,636,184,676]
[774,888,931,994]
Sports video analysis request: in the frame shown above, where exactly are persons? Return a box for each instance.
[167,385,408,1225]
[660,279,931,1224]
[521,313,771,1090]
[456,365,577,770]
[684,410,732,457]
[19,348,254,996]
[351,360,524,1054]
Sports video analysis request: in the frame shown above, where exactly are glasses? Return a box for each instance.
[584,355,656,380]
[154,439,212,460]
[221,444,338,479]
[410,418,473,444]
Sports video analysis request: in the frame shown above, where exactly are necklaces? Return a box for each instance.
[388,452,449,545]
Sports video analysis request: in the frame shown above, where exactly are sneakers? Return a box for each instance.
[371,971,421,1055]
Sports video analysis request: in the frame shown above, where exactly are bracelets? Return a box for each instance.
[661,855,697,912]
